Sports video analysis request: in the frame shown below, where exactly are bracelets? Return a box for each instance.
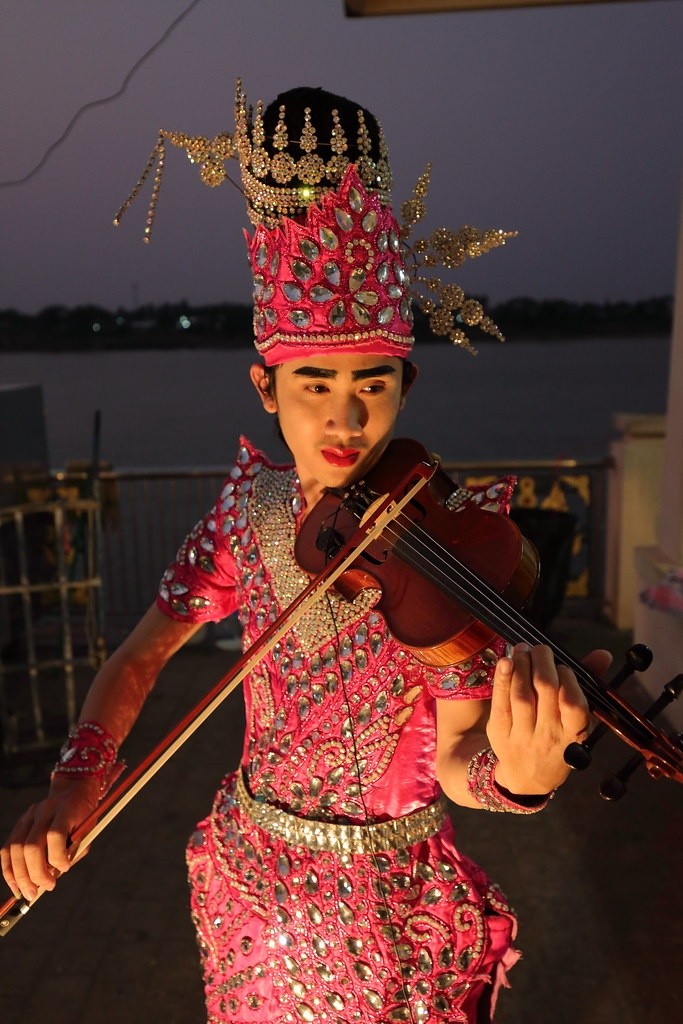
[467,747,556,815]
[51,719,125,800]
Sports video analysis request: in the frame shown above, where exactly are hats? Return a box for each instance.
[114,76,521,369]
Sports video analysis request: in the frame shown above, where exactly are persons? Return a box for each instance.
[1,80,613,1023]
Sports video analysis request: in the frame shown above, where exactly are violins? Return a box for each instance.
[293,436,682,815]
